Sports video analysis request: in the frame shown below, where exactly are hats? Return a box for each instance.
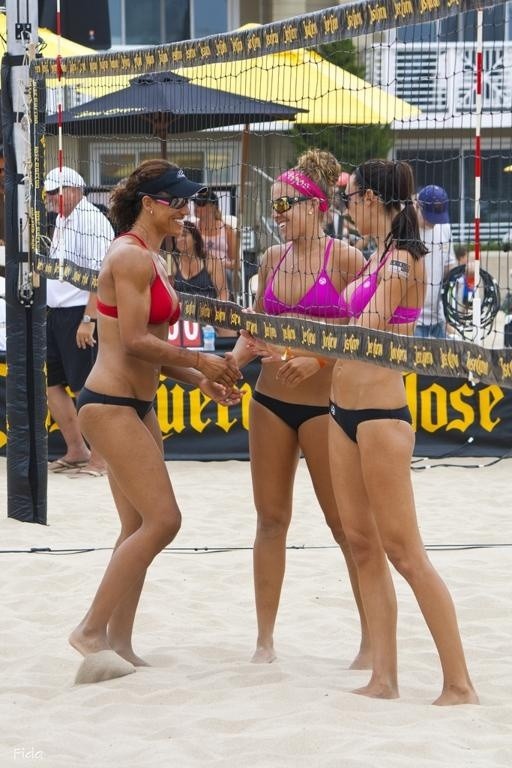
[43,167,85,192]
[134,165,208,197]
[419,184,451,223]
[194,185,218,207]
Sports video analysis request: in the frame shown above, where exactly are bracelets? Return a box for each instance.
[193,350,201,369]
[313,356,326,371]
[281,345,294,360]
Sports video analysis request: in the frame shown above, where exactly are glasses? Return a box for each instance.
[151,195,189,209]
[270,195,307,215]
[340,190,364,207]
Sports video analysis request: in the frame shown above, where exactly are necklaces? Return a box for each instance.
[290,241,323,269]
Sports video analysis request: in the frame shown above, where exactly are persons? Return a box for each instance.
[160,217,237,336]
[410,183,461,338]
[40,167,117,480]
[62,153,246,673]
[238,159,486,708]
[187,183,240,301]
[321,169,379,260]
[202,145,387,669]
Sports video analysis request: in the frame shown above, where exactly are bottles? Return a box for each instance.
[202,324,216,350]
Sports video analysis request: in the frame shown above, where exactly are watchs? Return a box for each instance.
[79,314,98,323]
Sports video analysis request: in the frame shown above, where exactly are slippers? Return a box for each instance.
[49,459,105,478]
